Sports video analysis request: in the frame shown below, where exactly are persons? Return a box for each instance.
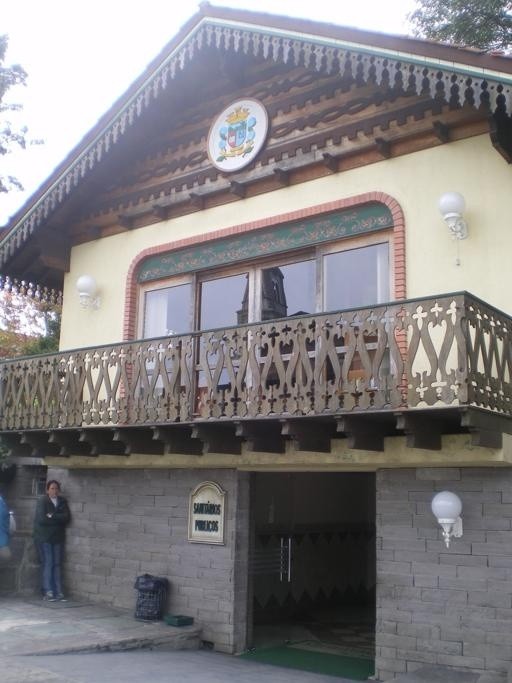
[1,497,11,558]
[33,480,73,602]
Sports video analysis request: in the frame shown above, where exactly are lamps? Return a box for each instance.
[74,274,101,310]
[437,193,468,242]
[430,489,464,545]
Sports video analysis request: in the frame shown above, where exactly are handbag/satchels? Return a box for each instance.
[134,574,167,592]
[134,590,161,620]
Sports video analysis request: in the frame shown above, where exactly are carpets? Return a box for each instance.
[239,643,376,680]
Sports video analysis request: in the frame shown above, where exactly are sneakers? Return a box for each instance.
[42,591,55,601]
[56,593,66,601]
